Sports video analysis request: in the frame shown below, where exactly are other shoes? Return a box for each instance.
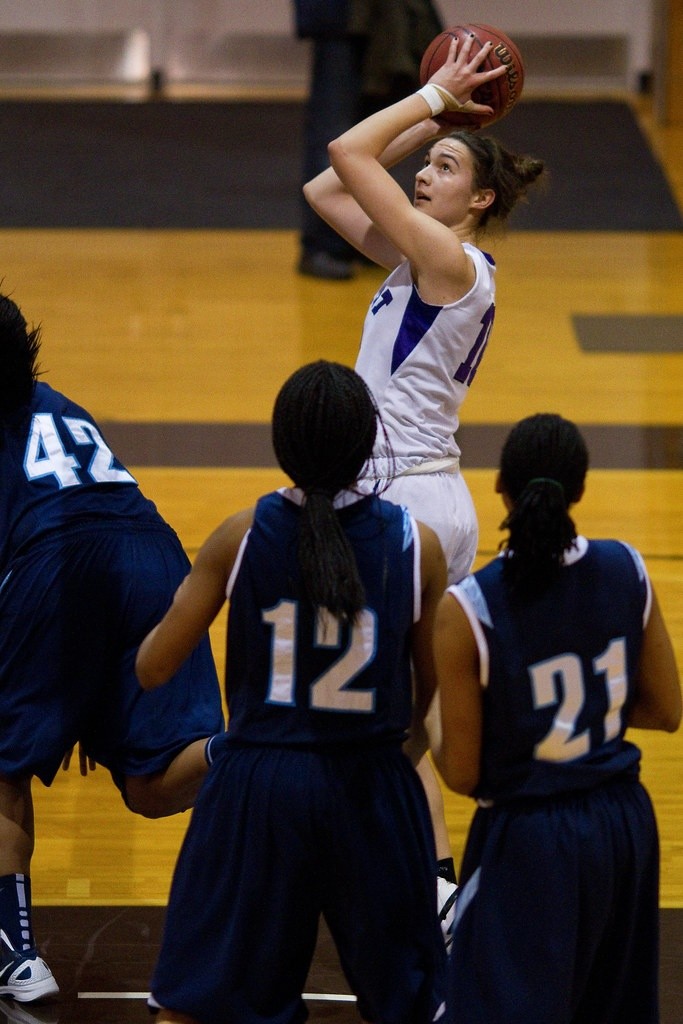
[298,248,357,279]
[432,875,464,949]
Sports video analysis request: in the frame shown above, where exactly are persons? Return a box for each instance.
[300,32,544,779]
[129,362,470,1023]
[415,413,683,1023]
[288,1,450,282]
[0,288,234,1007]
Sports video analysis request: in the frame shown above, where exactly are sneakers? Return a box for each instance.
[0,955,59,1004]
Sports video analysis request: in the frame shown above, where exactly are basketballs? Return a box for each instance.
[419,23,526,132]
